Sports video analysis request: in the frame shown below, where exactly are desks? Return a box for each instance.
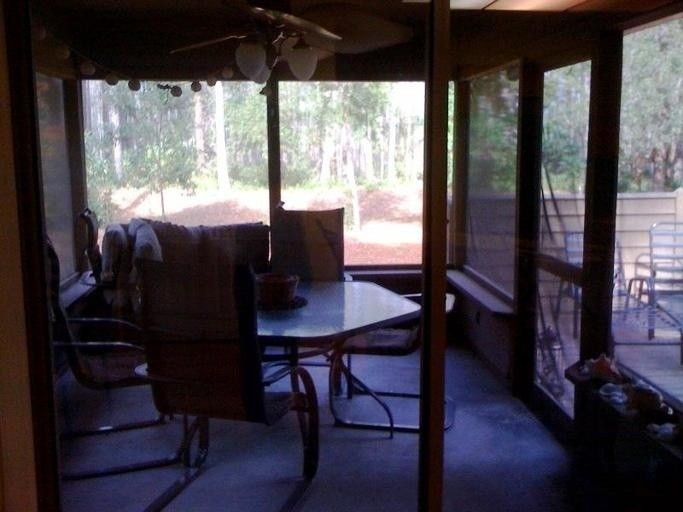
[252,279,422,441]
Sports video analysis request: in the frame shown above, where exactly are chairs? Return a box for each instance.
[137,219,320,478]
[331,288,459,430]
[52,240,186,480]
[73,207,144,353]
[624,220,683,342]
[272,203,359,401]
[553,230,638,338]
[103,222,182,420]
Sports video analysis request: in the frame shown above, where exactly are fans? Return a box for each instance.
[164,0,343,55]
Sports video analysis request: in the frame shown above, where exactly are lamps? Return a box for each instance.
[235,21,319,82]
